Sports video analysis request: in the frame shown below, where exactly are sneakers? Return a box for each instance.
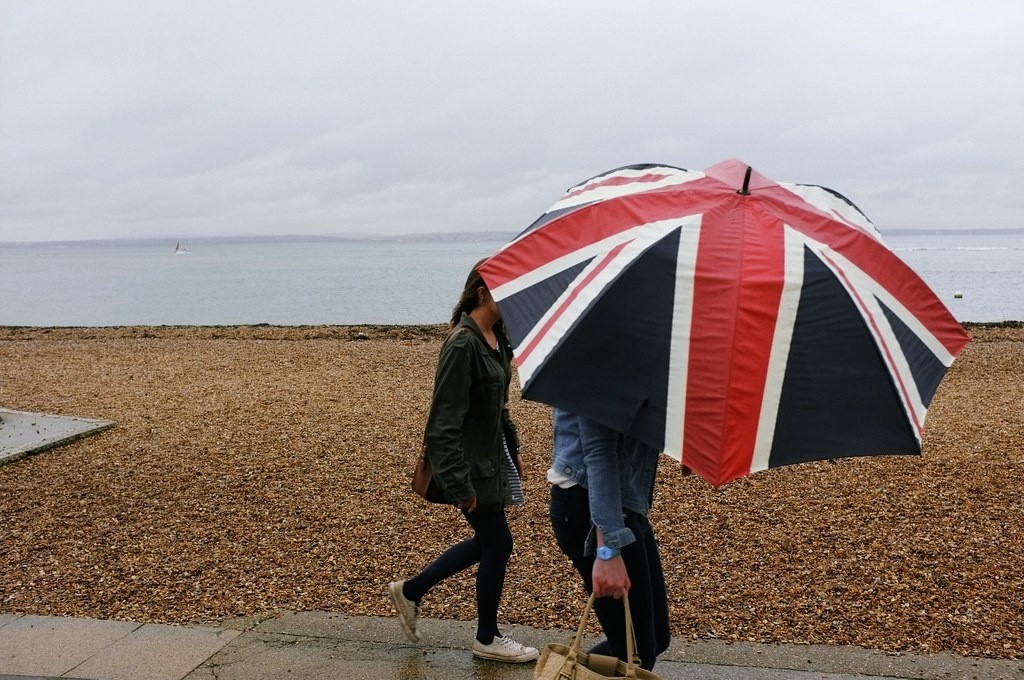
[388,580,422,643]
[472,634,540,663]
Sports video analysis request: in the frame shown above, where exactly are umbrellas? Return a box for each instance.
[474,156,973,490]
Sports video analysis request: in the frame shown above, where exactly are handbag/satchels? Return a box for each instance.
[410,445,453,506]
[532,587,664,680]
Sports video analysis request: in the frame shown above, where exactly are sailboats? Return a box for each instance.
[175,241,189,254]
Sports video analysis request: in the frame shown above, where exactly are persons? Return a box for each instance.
[546,406,671,672]
[387,256,539,664]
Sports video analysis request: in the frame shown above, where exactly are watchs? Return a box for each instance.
[595,547,623,561]
